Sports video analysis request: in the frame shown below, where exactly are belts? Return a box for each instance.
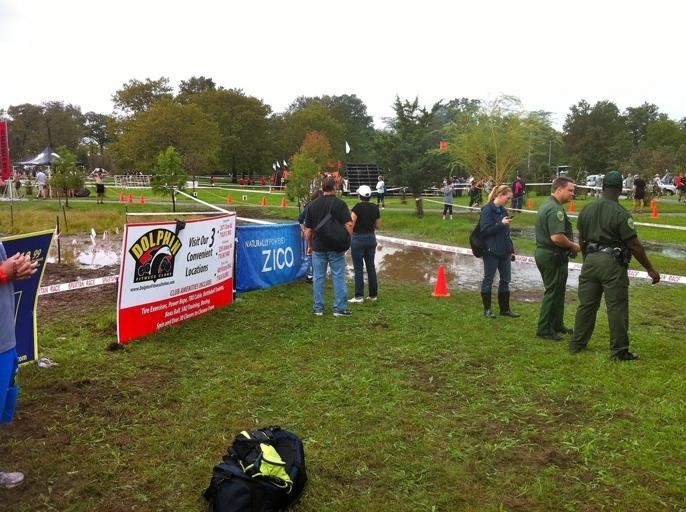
[598,243,626,255]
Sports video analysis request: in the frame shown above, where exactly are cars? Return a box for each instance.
[584,172,677,197]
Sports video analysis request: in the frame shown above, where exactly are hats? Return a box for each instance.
[356,185,372,197]
[603,171,623,187]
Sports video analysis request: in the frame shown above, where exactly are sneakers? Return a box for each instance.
[313,307,323,315]
[536,329,564,340]
[365,295,378,301]
[557,326,573,334]
[347,296,364,304]
[333,310,352,317]
[612,352,641,360]
[570,343,586,354]
[0,471,23,489]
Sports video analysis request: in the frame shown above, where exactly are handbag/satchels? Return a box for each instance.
[314,214,351,252]
[470,225,487,258]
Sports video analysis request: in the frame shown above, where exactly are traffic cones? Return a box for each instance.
[225,189,288,208]
[116,190,146,206]
[529,199,533,208]
[570,201,575,211]
[650,200,658,217]
[433,265,452,300]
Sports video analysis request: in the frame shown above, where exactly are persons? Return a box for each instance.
[569,171,660,361]
[441,175,466,197]
[376,176,385,208]
[299,191,324,281]
[626,173,646,214]
[653,174,685,202]
[535,177,581,339]
[468,175,498,206]
[510,175,525,213]
[209,176,217,187]
[470,185,521,317]
[261,175,266,186]
[0,240,40,487]
[347,185,382,302]
[12,168,47,199]
[305,177,353,317]
[89,168,110,204]
[442,182,455,219]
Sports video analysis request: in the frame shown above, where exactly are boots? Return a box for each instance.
[498,289,521,317]
[480,292,497,318]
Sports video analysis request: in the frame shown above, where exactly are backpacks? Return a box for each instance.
[203,424,307,511]
